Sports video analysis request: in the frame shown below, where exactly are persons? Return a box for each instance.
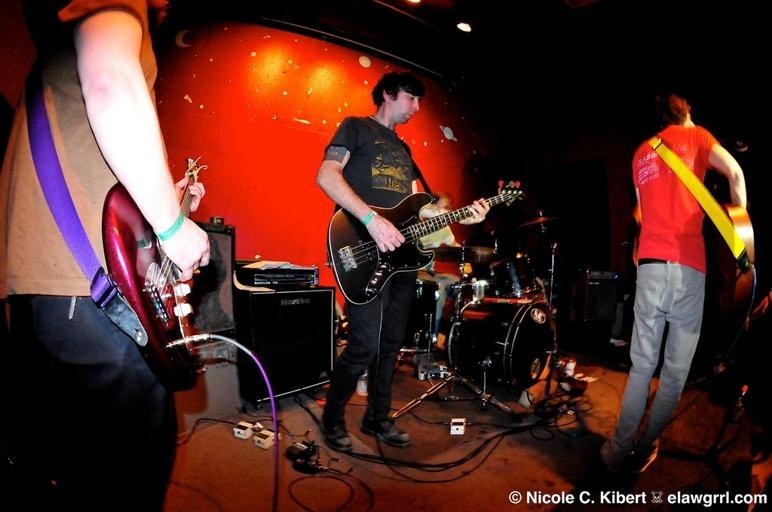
[601,95,749,466]
[315,71,490,453]
[0,0,212,511]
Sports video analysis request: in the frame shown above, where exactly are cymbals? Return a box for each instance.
[438,242,489,254]
[518,216,556,228]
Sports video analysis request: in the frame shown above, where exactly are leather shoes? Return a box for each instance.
[361,413,411,448]
[321,417,352,452]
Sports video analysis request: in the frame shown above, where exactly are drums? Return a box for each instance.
[449,280,494,296]
[446,298,557,392]
[399,280,439,353]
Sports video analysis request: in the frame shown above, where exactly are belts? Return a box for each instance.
[638,257,667,266]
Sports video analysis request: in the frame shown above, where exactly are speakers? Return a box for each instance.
[235,287,335,402]
[586,271,618,321]
[189,221,237,344]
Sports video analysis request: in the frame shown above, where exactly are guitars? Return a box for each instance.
[327,179,526,306]
[102,156,208,391]
[703,203,756,352]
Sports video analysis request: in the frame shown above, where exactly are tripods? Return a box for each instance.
[393,241,514,421]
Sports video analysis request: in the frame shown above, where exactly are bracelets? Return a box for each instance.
[158,210,184,241]
[361,211,378,227]
